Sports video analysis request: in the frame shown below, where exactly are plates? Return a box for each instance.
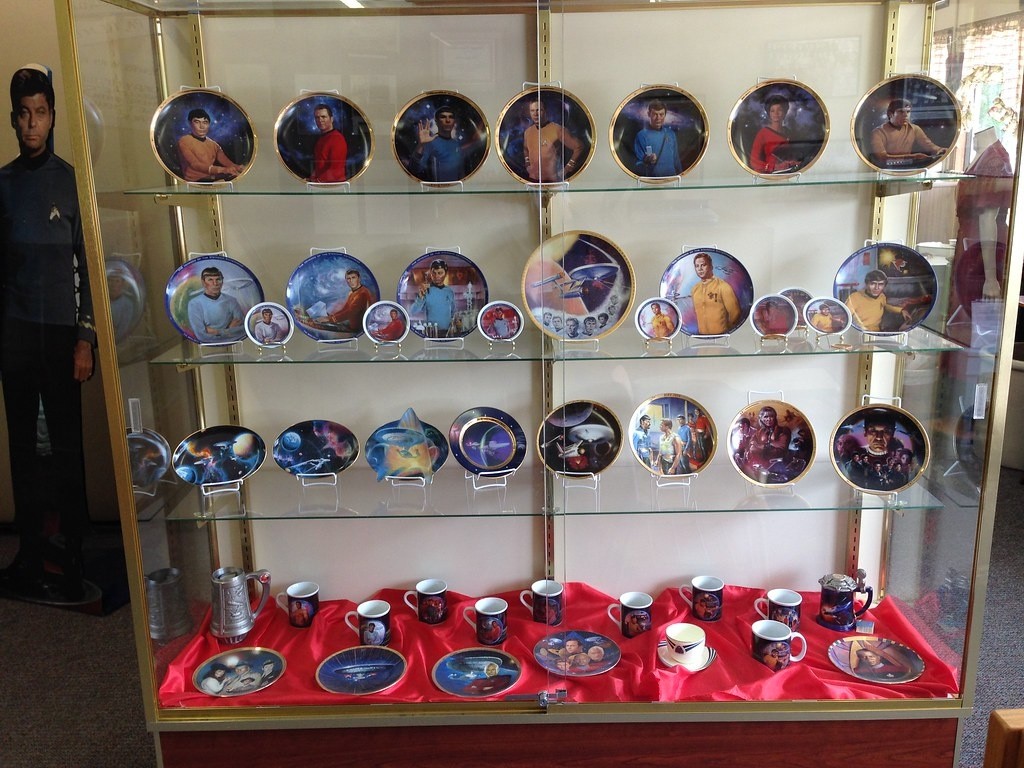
[191,647,287,697]
[315,645,408,696]
[533,630,621,677]
[431,647,523,699]
[132,72,963,493]
[656,639,717,674]
[828,636,926,685]
[105,258,148,346]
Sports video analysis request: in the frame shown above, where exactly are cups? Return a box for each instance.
[403,579,448,625]
[665,623,706,664]
[749,620,807,672]
[817,573,874,632]
[210,566,272,644]
[344,599,391,647]
[607,592,654,638]
[520,579,565,627]
[143,567,194,644]
[753,588,803,633]
[463,597,509,647]
[276,582,320,629]
[679,575,725,623]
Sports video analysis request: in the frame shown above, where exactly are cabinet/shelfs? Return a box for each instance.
[51,0,1024,768]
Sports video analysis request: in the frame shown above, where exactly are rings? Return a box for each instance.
[88,373,91,375]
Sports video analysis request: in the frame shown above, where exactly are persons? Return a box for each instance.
[928,127,1015,496]
[0,68,97,599]
